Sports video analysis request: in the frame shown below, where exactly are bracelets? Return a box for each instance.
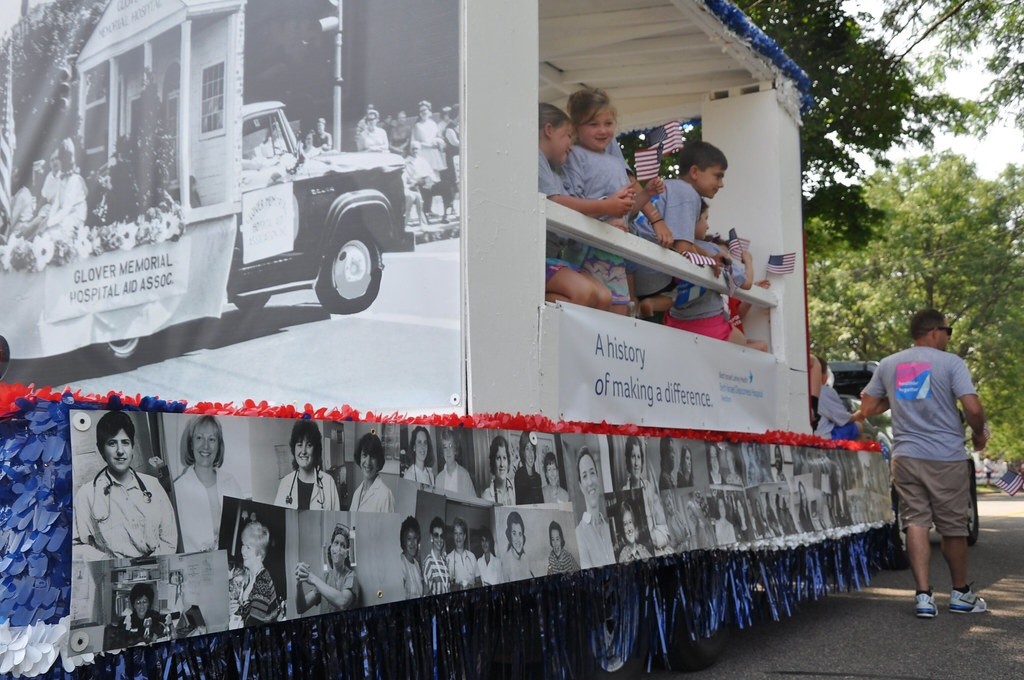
[651,218,664,226]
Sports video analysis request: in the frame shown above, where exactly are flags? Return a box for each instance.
[767,252,796,275]
[634,143,664,181]
[646,121,683,156]
[728,228,750,262]
[995,469,1023,496]
[681,250,716,267]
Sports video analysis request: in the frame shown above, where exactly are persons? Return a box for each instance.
[71,413,854,648]
[860,309,988,619]
[984,453,994,489]
[539,88,773,354]
[810,354,864,440]
[298,100,465,227]
[5,136,91,268]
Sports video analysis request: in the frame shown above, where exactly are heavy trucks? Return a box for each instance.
[547,360,979,680]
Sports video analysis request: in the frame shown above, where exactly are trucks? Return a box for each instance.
[0,98,416,376]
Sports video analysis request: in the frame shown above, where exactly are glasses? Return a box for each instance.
[368,117,376,121]
[433,532,444,539]
[928,326,953,336]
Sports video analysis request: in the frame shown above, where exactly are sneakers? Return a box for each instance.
[915,586,938,617]
[948,581,987,613]
